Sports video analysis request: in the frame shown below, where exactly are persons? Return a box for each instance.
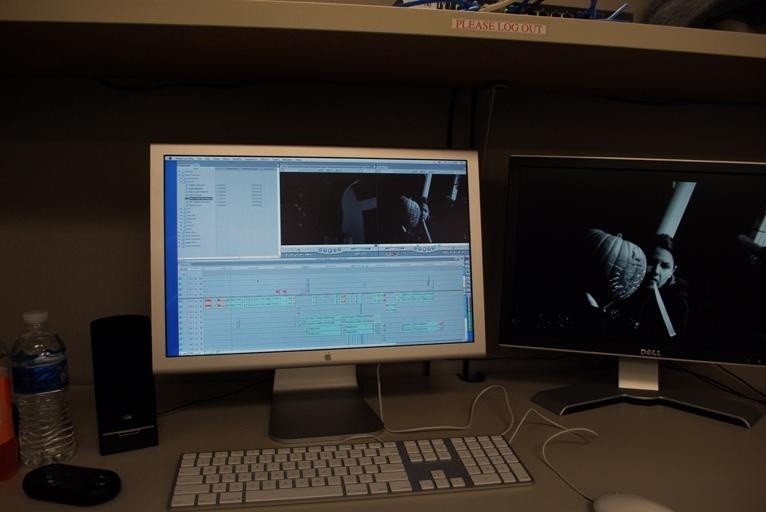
[399,197,432,243]
[586,235,689,358]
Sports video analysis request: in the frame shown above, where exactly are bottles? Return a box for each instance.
[11,309,76,468]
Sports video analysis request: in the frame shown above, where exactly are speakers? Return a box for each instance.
[92,314,159,457]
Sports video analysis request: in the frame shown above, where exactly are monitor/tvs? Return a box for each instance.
[498,152,765,432]
[145,141,489,439]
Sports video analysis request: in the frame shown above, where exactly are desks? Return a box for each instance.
[29,379,765,512]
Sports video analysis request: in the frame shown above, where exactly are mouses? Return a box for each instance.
[590,488,673,512]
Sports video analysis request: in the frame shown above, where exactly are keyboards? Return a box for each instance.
[161,434,535,512]
